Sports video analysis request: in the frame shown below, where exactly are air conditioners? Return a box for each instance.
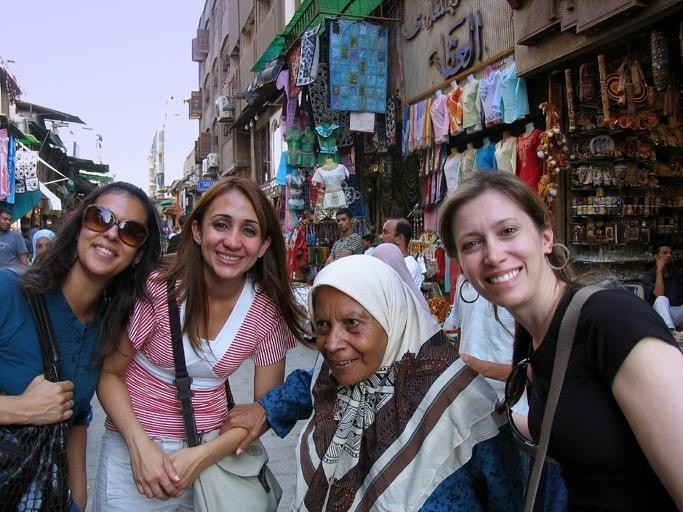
[205,153,218,171]
[201,158,216,177]
[213,94,233,123]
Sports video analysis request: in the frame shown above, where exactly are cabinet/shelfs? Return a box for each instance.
[557,71,683,269]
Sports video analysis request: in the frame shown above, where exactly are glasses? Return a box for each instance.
[501,356,546,455]
[82,201,150,250]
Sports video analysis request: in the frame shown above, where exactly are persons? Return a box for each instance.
[427,53,542,182]
[313,159,351,212]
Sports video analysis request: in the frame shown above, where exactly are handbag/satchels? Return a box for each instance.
[189,426,285,512]
[0,382,79,512]
